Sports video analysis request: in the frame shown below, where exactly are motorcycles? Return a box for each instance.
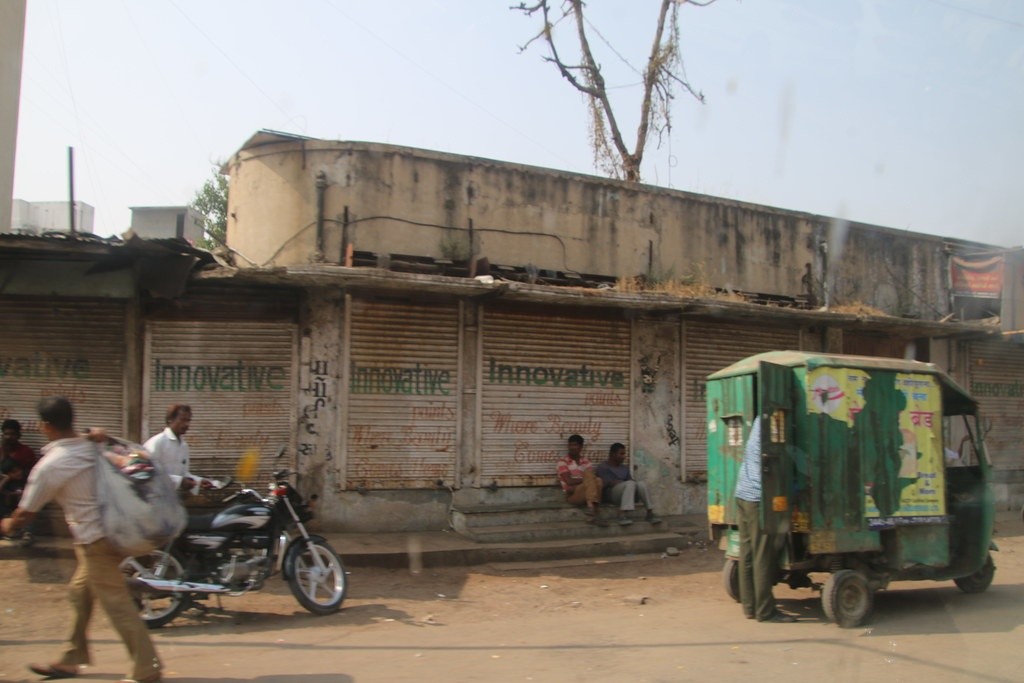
[698,352,999,630]
[112,444,352,633]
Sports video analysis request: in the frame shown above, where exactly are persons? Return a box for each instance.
[594,443,662,526]
[0,418,38,546]
[556,434,603,516]
[0,395,167,683]
[143,405,213,611]
[734,415,797,623]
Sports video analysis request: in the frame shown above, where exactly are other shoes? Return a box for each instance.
[121,672,160,683]
[30,663,75,677]
[616,516,632,525]
[585,509,596,517]
[746,609,795,622]
[645,514,662,524]
[591,517,608,527]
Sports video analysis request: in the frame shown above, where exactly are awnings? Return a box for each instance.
[193,267,1002,340]
